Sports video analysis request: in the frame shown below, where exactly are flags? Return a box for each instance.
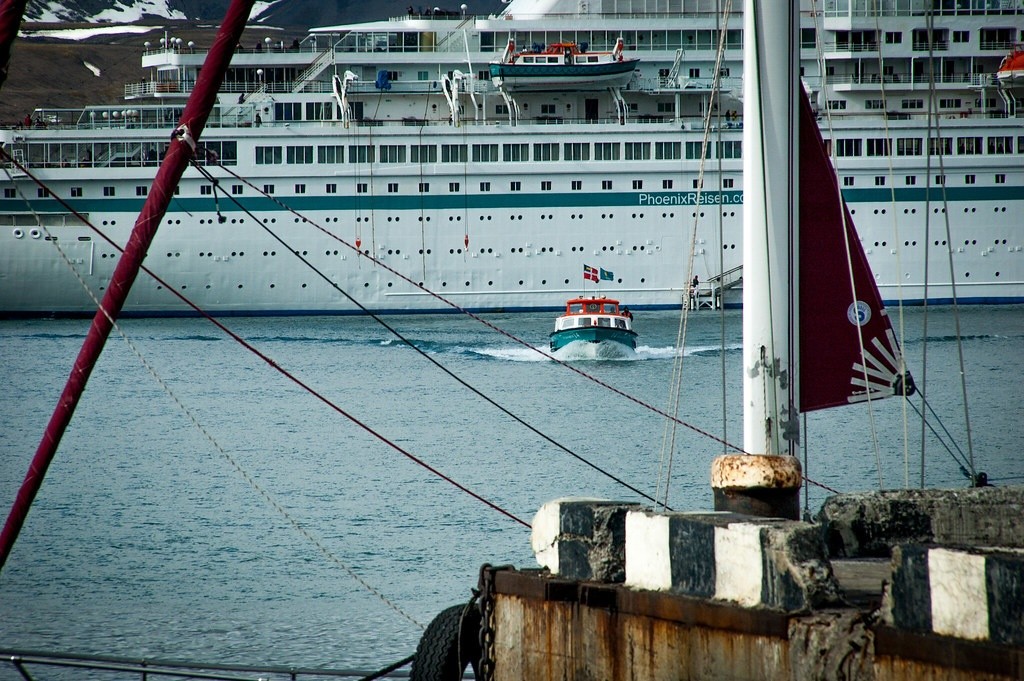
[583,264,599,281]
[600,267,613,281]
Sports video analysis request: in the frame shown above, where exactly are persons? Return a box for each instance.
[693,275,699,288]
[620,305,633,328]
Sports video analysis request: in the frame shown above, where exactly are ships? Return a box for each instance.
[0,0,1024,319]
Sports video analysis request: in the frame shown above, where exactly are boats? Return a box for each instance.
[548,294,639,356]
[489,37,641,92]
[995,48,1024,82]
